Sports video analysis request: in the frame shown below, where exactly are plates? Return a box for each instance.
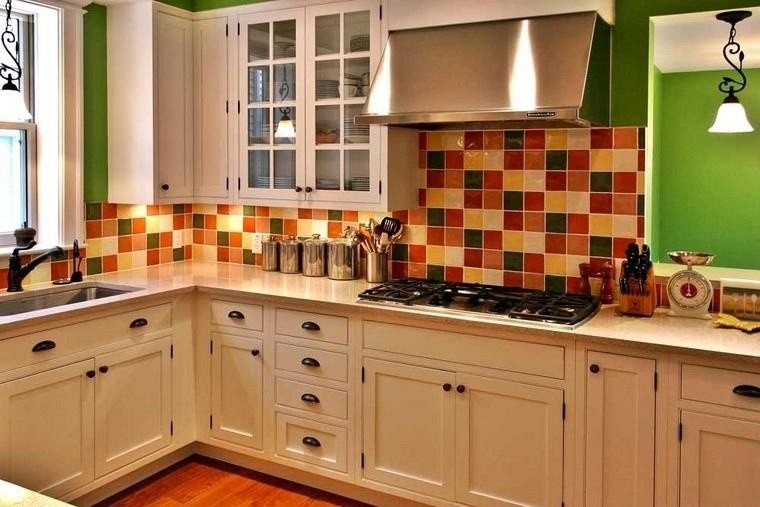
[351,175,369,190]
[316,178,340,189]
[257,175,295,187]
[261,81,295,100]
[315,79,338,99]
[344,116,369,142]
[349,32,369,51]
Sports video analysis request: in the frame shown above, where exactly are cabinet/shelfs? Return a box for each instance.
[104,1,195,212]
[231,0,421,215]
[567,329,655,507]
[195,282,270,485]
[348,302,565,506]
[267,293,355,507]
[191,7,240,211]
[1,286,198,507]
[655,344,759,505]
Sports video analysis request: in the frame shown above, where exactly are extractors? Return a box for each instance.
[353,7,609,127]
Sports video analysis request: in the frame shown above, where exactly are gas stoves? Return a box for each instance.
[356,274,603,330]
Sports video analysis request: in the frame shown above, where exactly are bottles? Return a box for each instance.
[261,233,362,281]
[578,261,613,304]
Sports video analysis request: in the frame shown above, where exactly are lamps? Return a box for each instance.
[0,0,35,122]
[703,8,755,137]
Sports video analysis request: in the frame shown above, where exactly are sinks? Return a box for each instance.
[0,280,147,317]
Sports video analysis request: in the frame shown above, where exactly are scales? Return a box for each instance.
[666,251,716,320]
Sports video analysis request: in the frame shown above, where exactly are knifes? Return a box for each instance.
[620,241,653,296]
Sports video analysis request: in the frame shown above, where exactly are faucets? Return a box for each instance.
[7,240,67,292]
[71,239,81,282]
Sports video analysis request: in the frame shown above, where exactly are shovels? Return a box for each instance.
[381,217,401,239]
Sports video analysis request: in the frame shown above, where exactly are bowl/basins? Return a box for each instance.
[258,122,286,144]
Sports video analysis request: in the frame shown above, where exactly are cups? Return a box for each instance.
[365,252,389,282]
[337,72,367,97]
